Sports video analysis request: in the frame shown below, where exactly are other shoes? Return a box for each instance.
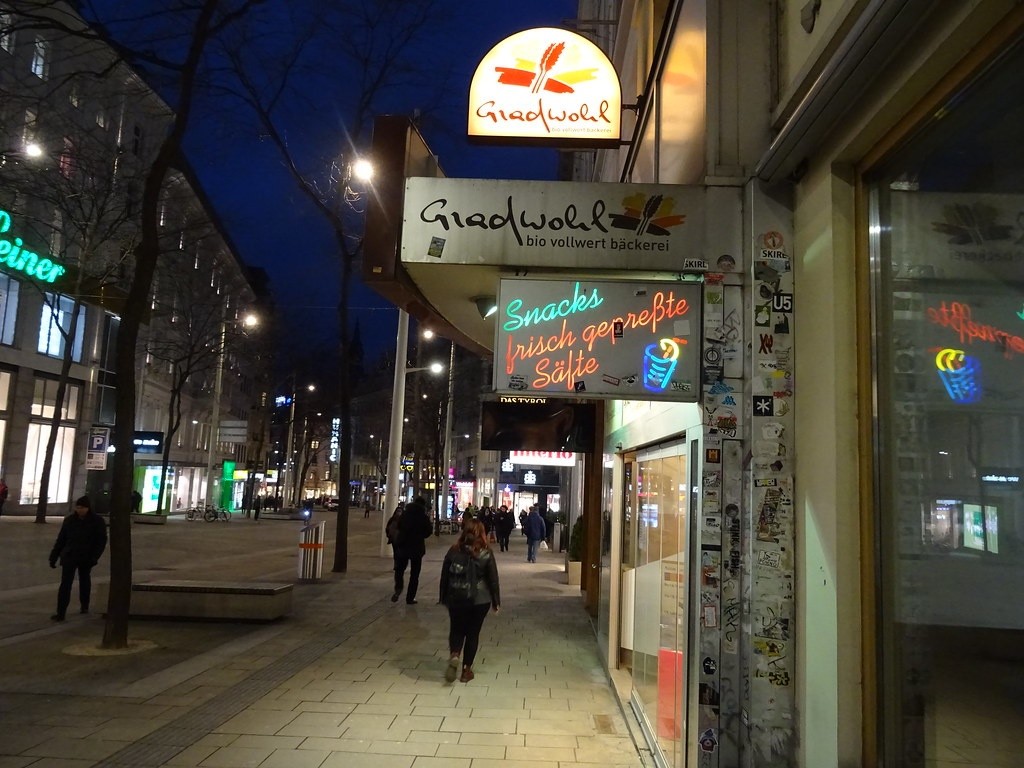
[50,614,65,621]
[500,549,504,552]
[444,655,459,683]
[406,599,418,604]
[527,558,536,564]
[391,594,398,602]
[460,669,474,683]
[80,609,88,614]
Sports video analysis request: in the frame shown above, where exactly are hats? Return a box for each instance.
[75,495,90,508]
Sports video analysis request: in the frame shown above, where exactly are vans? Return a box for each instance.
[321,495,338,511]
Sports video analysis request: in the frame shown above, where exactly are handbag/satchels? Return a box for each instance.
[446,542,479,602]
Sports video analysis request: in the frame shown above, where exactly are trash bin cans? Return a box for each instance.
[297,520,324,582]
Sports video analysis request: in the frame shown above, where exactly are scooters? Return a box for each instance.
[304,510,310,526]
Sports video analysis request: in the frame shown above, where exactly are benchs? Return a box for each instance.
[90,578,295,625]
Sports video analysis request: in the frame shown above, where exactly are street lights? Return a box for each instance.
[441,341,469,519]
[205,296,256,516]
[380,309,443,558]
[283,384,315,510]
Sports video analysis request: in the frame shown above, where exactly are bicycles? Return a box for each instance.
[185,501,231,523]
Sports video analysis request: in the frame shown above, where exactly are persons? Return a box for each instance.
[365,501,369,518]
[241,495,283,520]
[132,490,142,513]
[0,479,8,515]
[519,503,546,563]
[385,497,434,604]
[439,517,501,682]
[49,495,108,622]
[461,503,516,552]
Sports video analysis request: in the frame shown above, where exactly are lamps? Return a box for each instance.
[473,295,496,321]
